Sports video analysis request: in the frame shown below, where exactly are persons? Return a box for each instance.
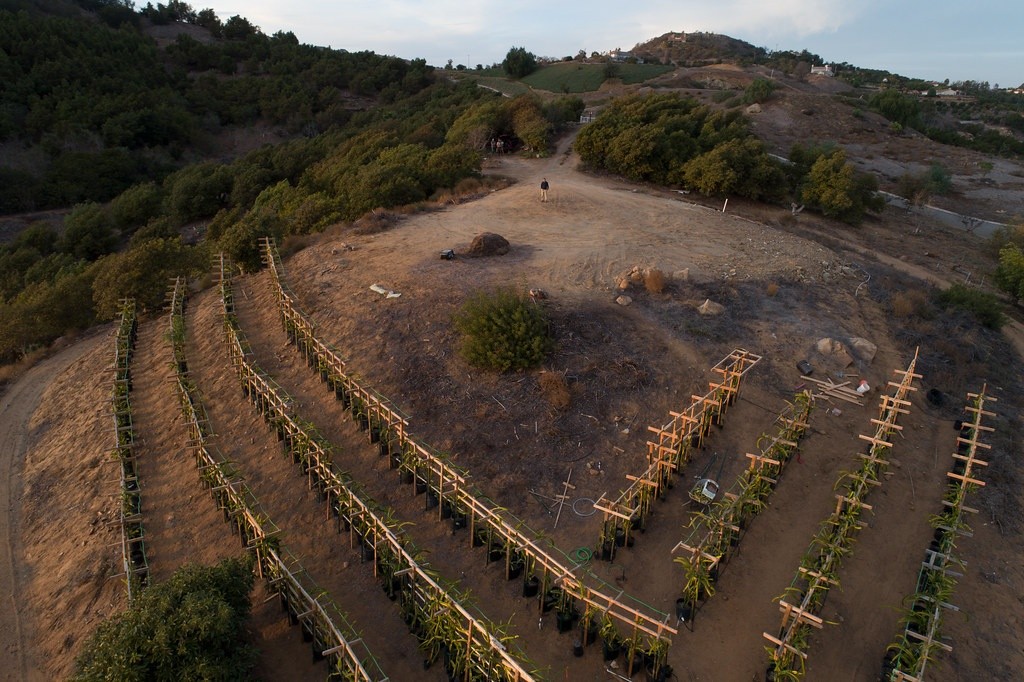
[491,135,514,158]
[540,178,549,202]
